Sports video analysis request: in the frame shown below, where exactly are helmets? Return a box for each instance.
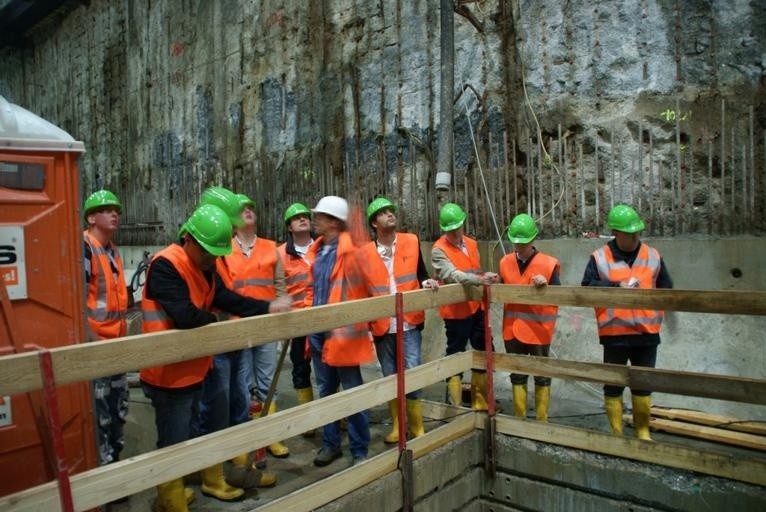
[438,203,467,231]
[235,193,257,210]
[84,190,123,215]
[507,213,539,244]
[177,204,234,256]
[608,204,645,233]
[311,195,349,224]
[366,198,398,223]
[199,186,246,227]
[283,203,309,224]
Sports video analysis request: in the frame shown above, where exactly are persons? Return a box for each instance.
[359,196,431,444]
[224,193,290,457]
[199,186,276,488]
[430,202,495,412]
[305,195,377,467]
[79,189,130,467]
[278,202,348,437]
[489,214,563,422]
[138,203,294,511]
[580,204,674,443]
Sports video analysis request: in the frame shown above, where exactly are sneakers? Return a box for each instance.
[313,444,345,466]
[355,455,369,467]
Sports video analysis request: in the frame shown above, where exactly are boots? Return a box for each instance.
[292,386,314,436]
[470,370,489,412]
[385,395,407,445]
[511,384,528,420]
[263,400,291,458]
[154,477,196,512]
[227,455,279,487]
[201,462,245,500]
[408,397,425,439]
[630,393,654,443]
[446,374,461,406]
[534,385,551,425]
[602,394,625,437]
